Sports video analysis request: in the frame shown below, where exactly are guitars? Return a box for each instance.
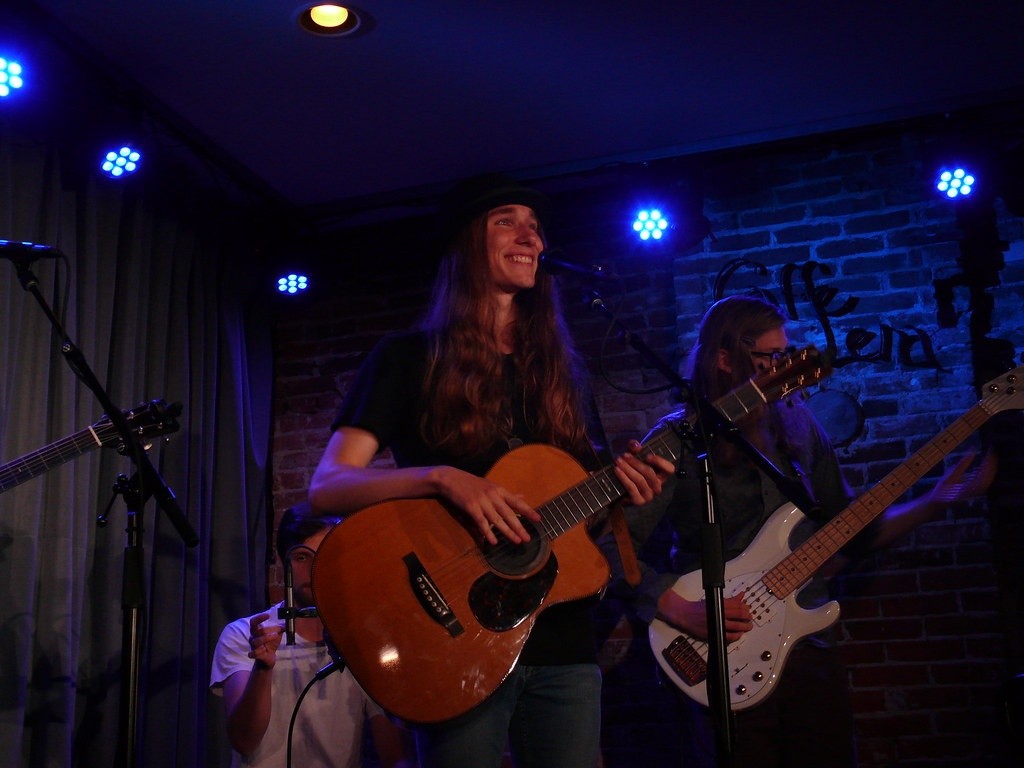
[308,343,834,736]
[647,365,1023,715]
[2,400,181,496]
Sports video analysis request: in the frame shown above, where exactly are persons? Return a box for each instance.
[208,505,409,767]
[306,179,676,768]
[594,294,997,768]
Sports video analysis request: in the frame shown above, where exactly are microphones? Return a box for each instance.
[0,240,63,258]
[285,559,295,646]
[537,248,608,273]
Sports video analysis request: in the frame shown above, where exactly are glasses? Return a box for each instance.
[751,346,796,367]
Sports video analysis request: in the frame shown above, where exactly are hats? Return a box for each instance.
[439,173,544,245]
